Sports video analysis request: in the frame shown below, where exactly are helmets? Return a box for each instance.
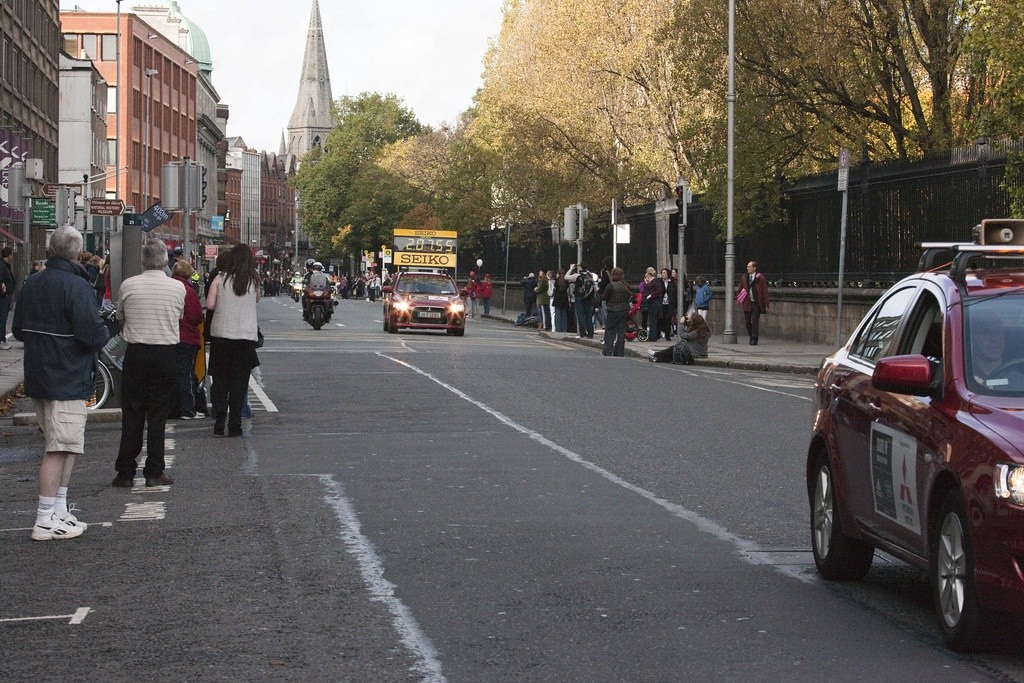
[305,259,315,269]
[312,262,322,271]
[295,272,300,276]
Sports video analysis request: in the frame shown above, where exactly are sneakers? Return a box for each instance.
[30,503,88,541]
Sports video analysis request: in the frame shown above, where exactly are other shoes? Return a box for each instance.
[0,340,12,350]
[228,426,243,437]
[749,337,758,345]
[146,473,173,486]
[180,411,206,419]
[111,471,134,486]
[647,349,657,362]
[212,423,226,435]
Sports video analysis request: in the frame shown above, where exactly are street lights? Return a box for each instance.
[143,68,160,251]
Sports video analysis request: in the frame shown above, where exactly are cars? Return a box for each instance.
[381,271,468,335]
[805,219,1023,652]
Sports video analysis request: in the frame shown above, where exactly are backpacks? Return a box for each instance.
[578,273,595,298]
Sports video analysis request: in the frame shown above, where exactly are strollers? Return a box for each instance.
[624,315,648,342]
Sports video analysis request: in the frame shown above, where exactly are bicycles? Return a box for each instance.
[86,307,120,411]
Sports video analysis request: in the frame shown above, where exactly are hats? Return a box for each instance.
[485,274,490,278]
[470,271,476,276]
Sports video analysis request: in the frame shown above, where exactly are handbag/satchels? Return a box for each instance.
[641,295,658,311]
[672,344,692,364]
[93,263,108,292]
[371,281,375,289]
[737,289,747,304]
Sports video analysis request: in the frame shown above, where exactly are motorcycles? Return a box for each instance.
[288,280,339,330]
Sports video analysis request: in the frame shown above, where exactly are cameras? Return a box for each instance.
[684,317,689,325]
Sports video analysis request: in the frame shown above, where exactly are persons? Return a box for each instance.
[13,224,108,538]
[302,258,335,318]
[601,267,631,357]
[519,256,690,341]
[460,271,481,319]
[0,246,17,350]
[259,269,303,297]
[28,243,264,437]
[332,272,398,302]
[734,261,769,345]
[480,274,493,315]
[693,275,712,322]
[648,312,711,363]
[933,307,1006,385]
[111,239,187,485]
